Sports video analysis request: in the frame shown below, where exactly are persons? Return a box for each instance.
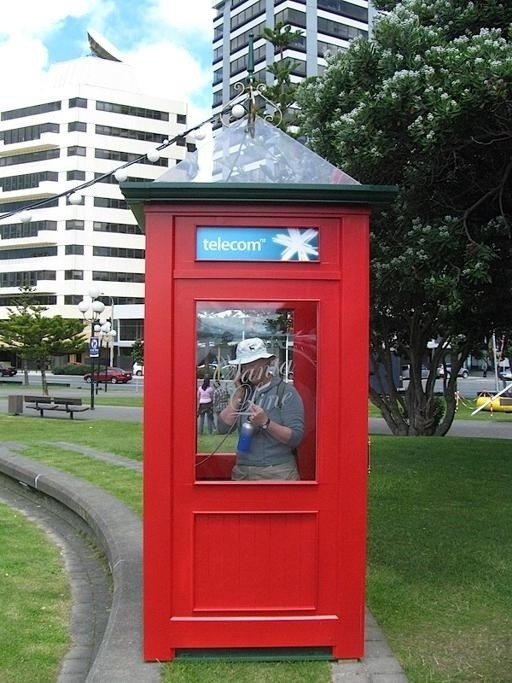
[217,335,307,482]
[196,373,215,435]
[212,380,229,416]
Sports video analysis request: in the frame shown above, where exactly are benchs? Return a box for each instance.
[25,396,58,417]
[0,381,22,384]
[54,398,89,420]
[47,383,70,387]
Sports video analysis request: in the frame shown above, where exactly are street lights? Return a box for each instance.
[78,287,105,410]
[95,319,116,393]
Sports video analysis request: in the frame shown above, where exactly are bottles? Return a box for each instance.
[235,419,255,453]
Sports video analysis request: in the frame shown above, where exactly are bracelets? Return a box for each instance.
[260,417,272,432]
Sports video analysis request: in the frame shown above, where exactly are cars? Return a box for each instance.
[83,367,133,383]
[499,370,512,382]
[438,362,469,379]
[401,364,427,381]
[133,361,144,376]
[0,364,17,377]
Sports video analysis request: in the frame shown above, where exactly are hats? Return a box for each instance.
[228,336,276,366]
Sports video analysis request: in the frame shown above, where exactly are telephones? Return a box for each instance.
[240,366,251,388]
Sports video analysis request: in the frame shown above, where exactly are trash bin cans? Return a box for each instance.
[8,396,23,416]
